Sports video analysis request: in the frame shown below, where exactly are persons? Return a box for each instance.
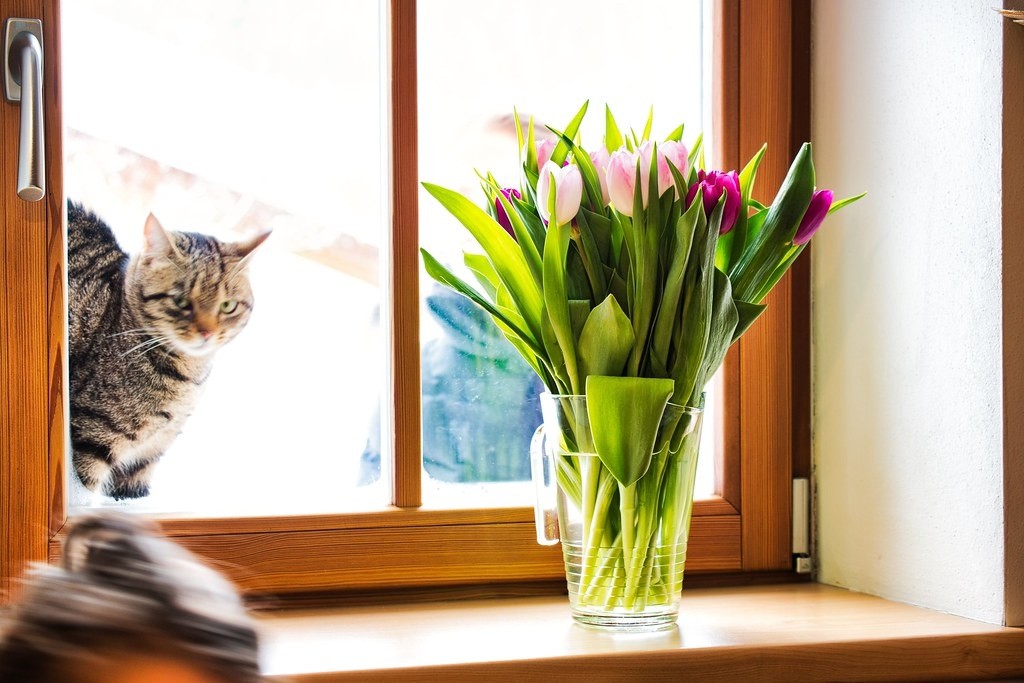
[359,111,622,489]
[0,508,261,683]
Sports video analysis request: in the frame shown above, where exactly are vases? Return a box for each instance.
[527,390,706,630]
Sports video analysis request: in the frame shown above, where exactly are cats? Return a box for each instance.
[67,197,274,500]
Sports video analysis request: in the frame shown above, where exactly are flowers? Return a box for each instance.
[418,99,870,609]
